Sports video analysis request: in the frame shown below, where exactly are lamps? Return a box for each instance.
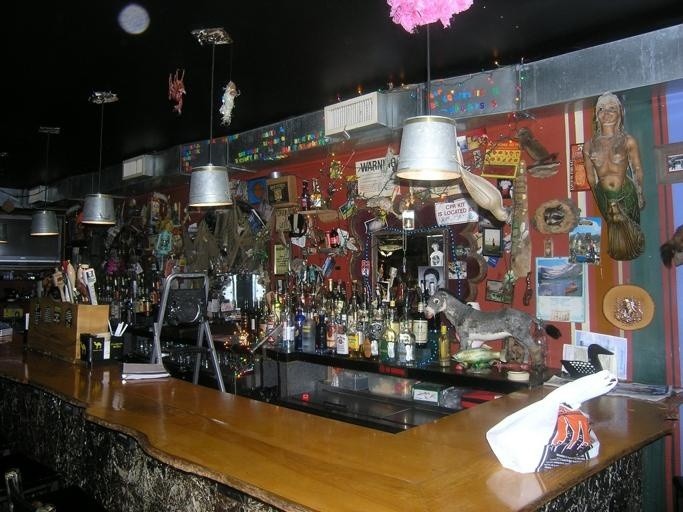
[30,125,61,237]
[78,90,118,225]
[395,23,462,182]
[188,26,232,207]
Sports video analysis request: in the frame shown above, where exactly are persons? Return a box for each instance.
[420,269,441,298]
[428,237,443,266]
[581,89,646,258]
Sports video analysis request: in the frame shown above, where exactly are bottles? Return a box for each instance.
[299,176,322,211]
[438,320,451,367]
[330,227,339,248]
[238,257,429,363]
[94,275,162,325]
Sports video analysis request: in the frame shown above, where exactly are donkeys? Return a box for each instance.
[424,288,563,373]
